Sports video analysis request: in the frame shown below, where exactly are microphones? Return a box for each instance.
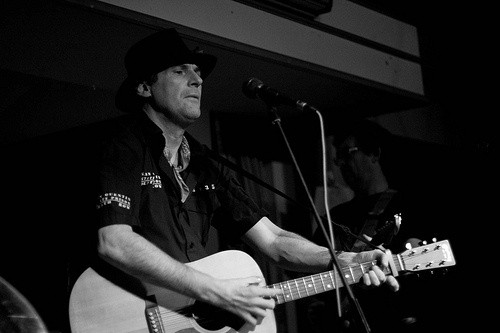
[242,77,317,113]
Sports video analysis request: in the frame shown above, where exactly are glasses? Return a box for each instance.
[334,146,369,164]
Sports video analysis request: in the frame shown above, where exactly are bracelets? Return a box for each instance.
[327,251,343,270]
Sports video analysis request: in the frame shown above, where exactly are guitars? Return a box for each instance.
[69,238,457,333]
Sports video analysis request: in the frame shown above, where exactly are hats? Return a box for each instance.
[113,27,218,113]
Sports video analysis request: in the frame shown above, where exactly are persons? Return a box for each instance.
[303,121,437,333]
[70,29,400,333]
